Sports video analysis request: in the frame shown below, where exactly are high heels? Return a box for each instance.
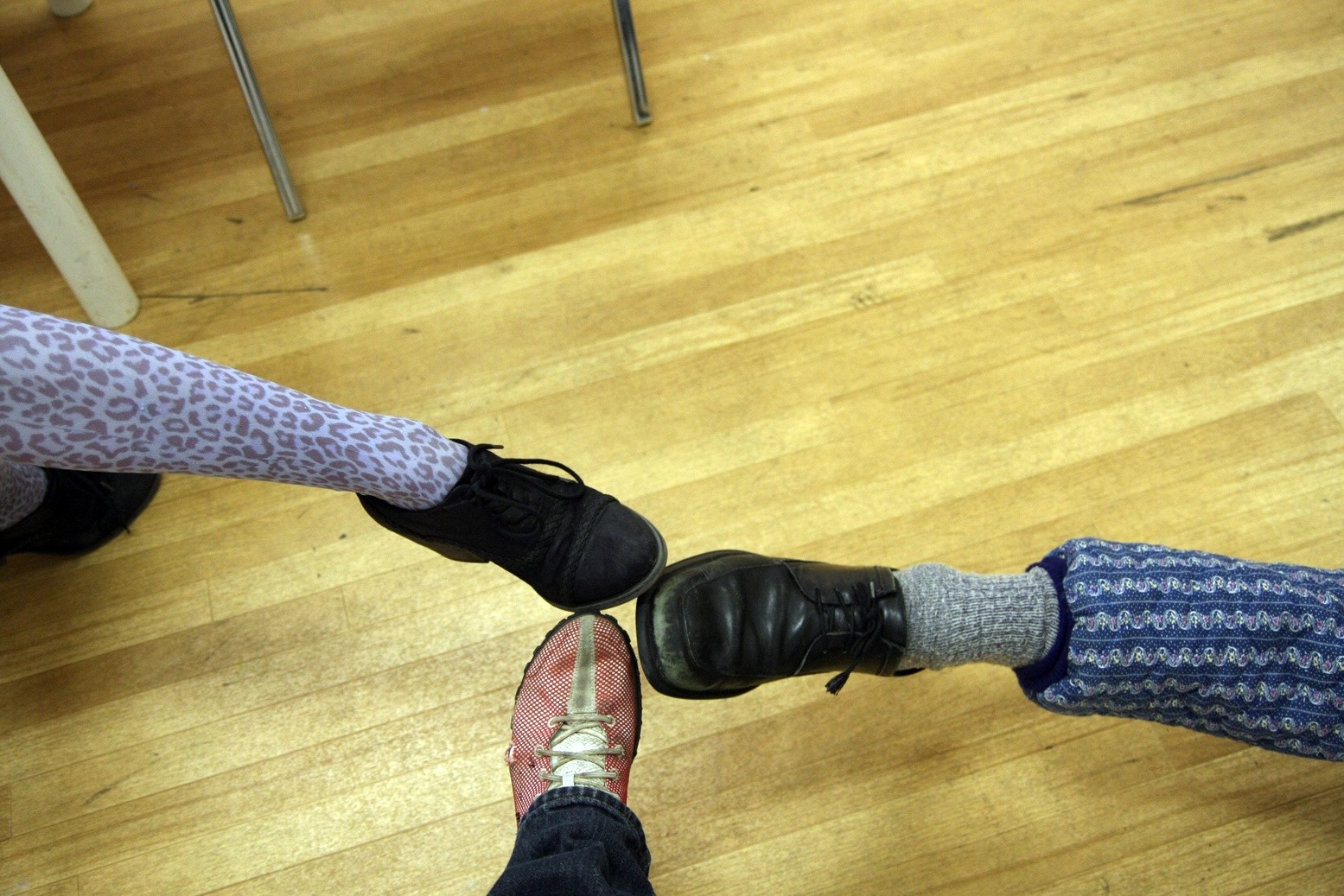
[352,418,666,612]
[3,468,160,566]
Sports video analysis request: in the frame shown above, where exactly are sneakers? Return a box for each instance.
[506,614,644,852]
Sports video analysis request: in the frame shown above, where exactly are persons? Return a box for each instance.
[0,303,668,612]
[636,536,1344,761]
[487,613,656,896]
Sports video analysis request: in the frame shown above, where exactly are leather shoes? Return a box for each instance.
[638,549,934,702]
